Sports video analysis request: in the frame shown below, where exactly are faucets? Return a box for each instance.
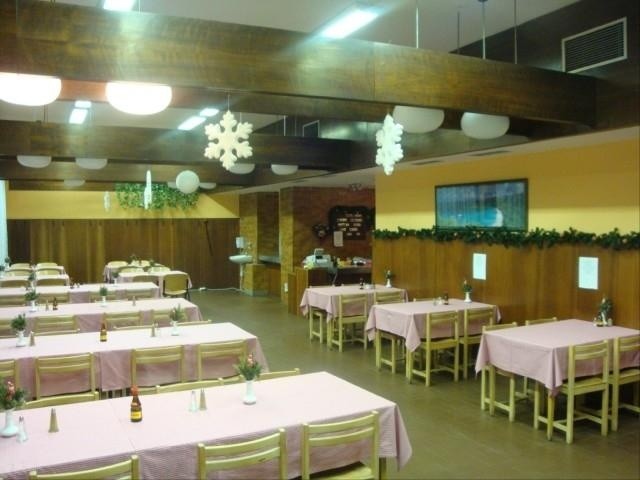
[240,248,248,255]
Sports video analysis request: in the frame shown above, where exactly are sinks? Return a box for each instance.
[228,255,253,265]
[259,255,280,266]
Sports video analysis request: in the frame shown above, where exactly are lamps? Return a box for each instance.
[392,0,510,140]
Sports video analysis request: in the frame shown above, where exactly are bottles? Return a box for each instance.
[443,291,449,304]
[45,296,58,311]
[432,295,443,306]
[100,322,106,341]
[189,390,197,412]
[333,255,337,268]
[49,407,59,432]
[359,277,364,289]
[130,390,143,422]
[16,415,29,442]
[199,387,207,410]
[132,296,136,306]
[593,312,614,327]
[150,322,159,337]
[29,330,35,347]
